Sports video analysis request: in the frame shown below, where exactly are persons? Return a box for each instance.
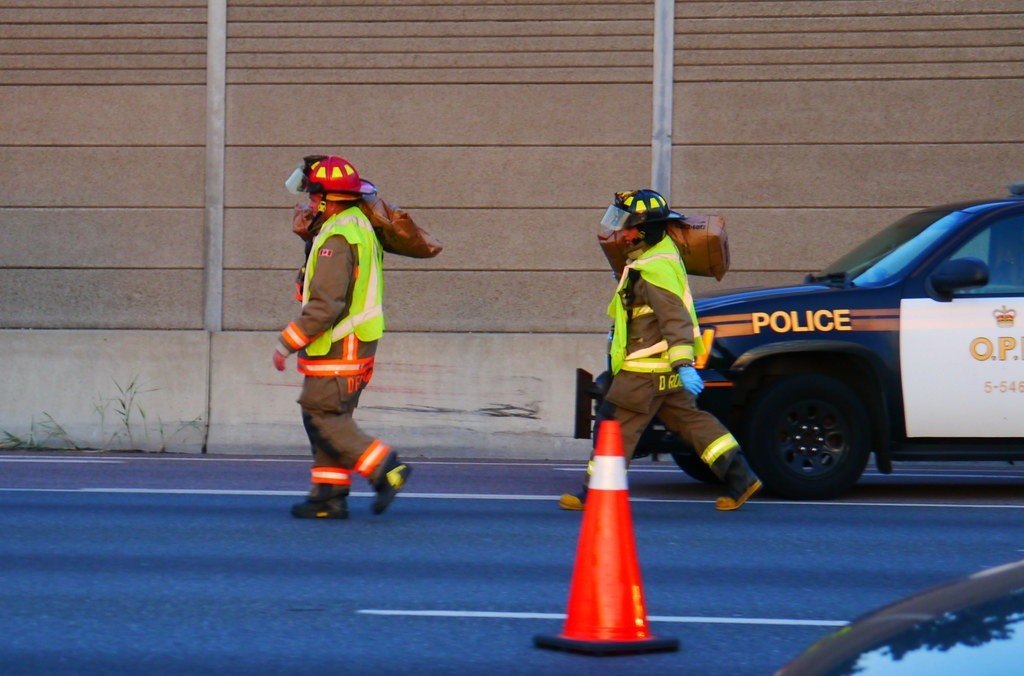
[273,155,413,519]
[558,189,761,511]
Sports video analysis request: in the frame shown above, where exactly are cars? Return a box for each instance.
[767,559,1024,676]
[570,181,1024,498]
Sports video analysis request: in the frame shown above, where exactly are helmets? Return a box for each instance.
[297,156,377,195]
[610,188,689,227]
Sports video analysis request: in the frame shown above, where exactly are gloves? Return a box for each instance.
[614,191,633,205]
[679,366,705,394]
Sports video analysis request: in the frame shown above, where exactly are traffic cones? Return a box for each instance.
[533,424,680,655]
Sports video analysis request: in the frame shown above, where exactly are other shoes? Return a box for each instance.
[716,452,764,510]
[558,484,590,510]
[292,491,350,521]
[370,451,414,515]
